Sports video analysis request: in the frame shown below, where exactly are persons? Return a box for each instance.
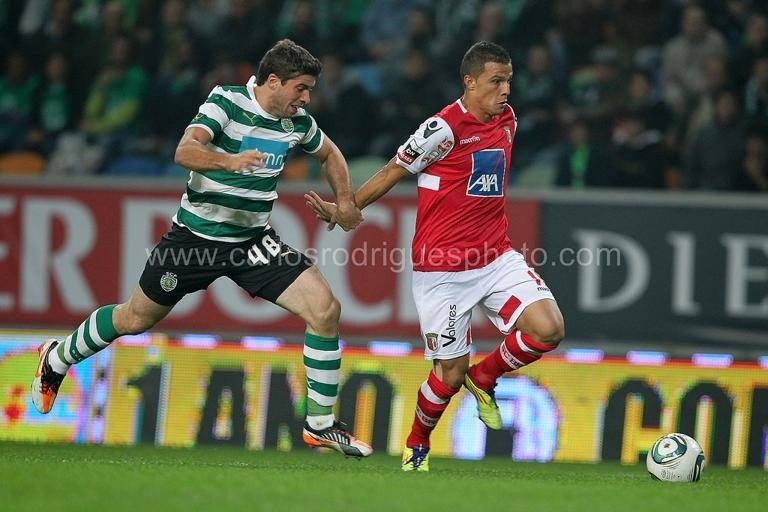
[29,40,374,459]
[303,40,567,474]
[0,0,768,206]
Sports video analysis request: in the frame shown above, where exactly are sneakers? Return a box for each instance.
[302,416,376,462]
[31,337,67,415]
[463,368,504,431]
[400,437,431,474]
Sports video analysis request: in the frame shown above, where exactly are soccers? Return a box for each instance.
[646,433,705,482]
